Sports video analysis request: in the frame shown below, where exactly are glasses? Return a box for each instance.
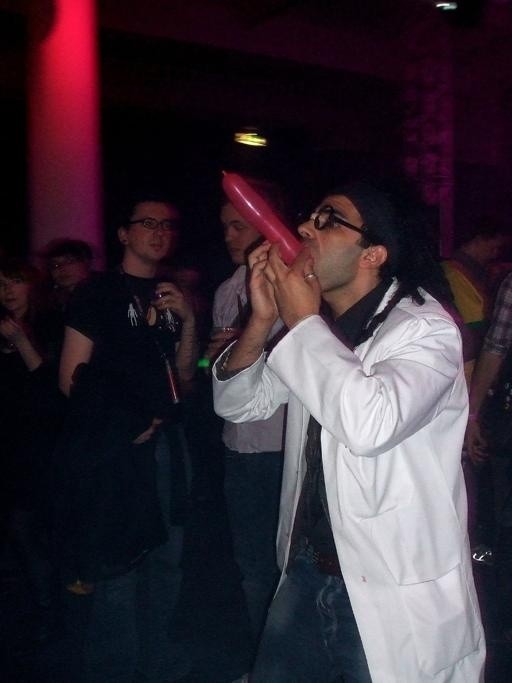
[125,217,177,232]
[313,203,371,245]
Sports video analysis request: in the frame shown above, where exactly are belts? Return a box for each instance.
[316,553,340,576]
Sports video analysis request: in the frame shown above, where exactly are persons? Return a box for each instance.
[212,155,489,682]
[0,189,289,683]
[417,203,511,541]
